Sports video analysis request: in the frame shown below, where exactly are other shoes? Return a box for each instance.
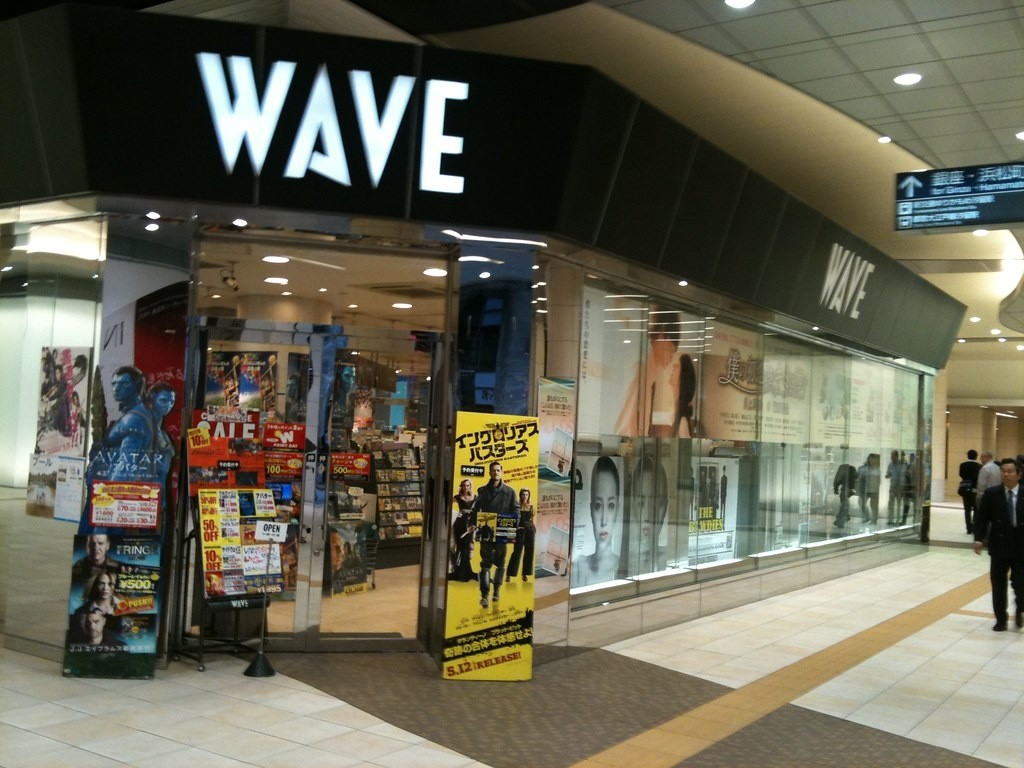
[861,517,870,523]
[1016,607,1024,628]
[833,519,844,529]
[993,622,1008,631]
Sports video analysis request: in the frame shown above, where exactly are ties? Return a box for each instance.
[1008,490,1014,527]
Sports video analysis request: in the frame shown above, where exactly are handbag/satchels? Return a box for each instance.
[957,479,972,497]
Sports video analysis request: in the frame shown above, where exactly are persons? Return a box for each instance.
[958,449,1024,632]
[689,465,728,531]
[67,365,175,650]
[616,303,696,439]
[833,450,915,528]
[571,456,621,588]
[628,457,667,575]
[451,462,536,609]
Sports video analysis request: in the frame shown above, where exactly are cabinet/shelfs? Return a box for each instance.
[366,446,427,570]
